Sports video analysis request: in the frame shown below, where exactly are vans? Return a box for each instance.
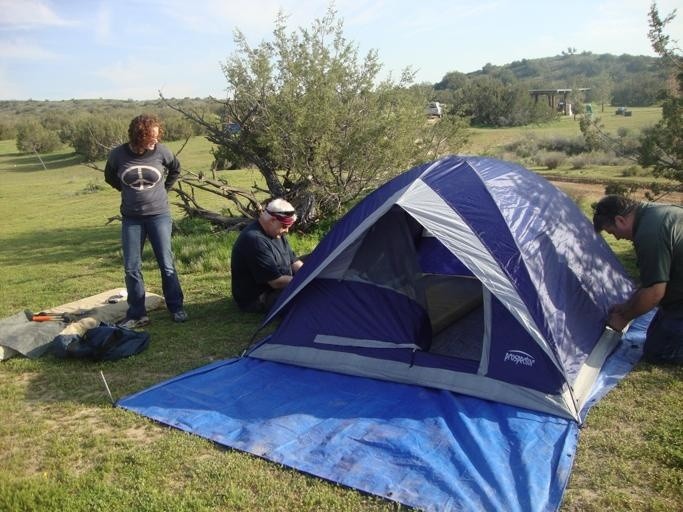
[425,101,443,118]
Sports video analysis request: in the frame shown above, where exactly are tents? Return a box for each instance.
[245,155,637,425]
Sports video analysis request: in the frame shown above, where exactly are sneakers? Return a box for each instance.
[118,316,150,329]
[171,309,188,322]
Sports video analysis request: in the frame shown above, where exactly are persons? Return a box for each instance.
[231,197,304,314]
[594,194,683,365]
[103,111,189,328]
